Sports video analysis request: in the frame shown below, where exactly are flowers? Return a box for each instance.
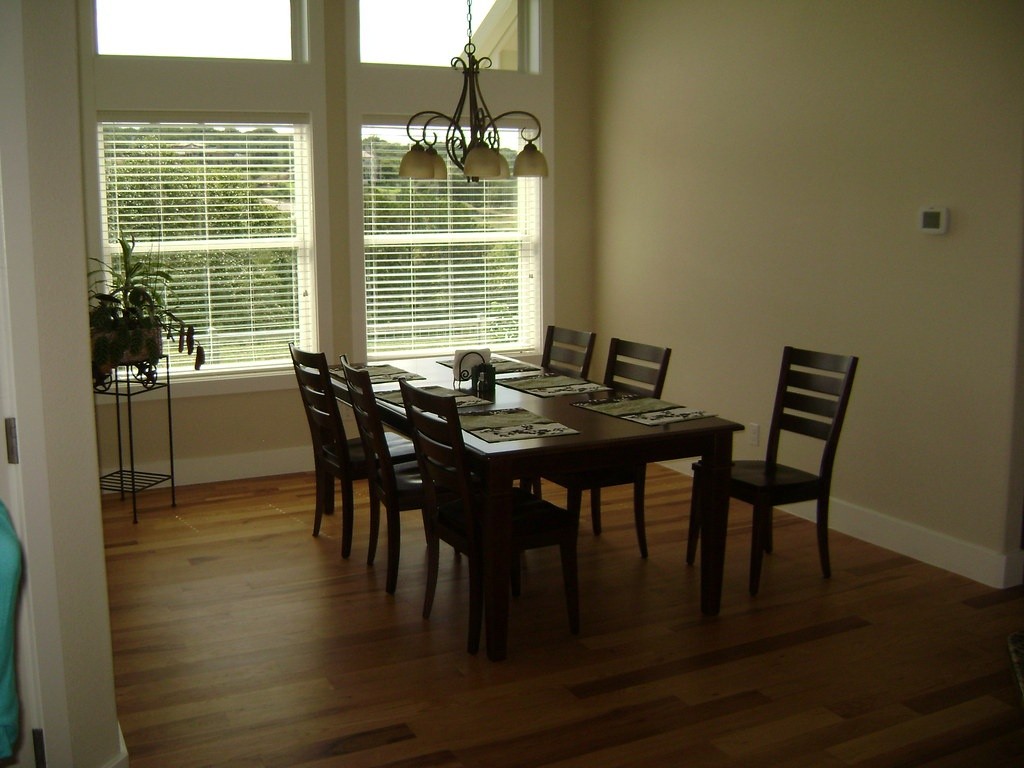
[88,229,205,371]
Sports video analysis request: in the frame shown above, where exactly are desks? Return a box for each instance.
[304,353,745,662]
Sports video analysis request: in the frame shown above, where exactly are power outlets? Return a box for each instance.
[748,422,759,445]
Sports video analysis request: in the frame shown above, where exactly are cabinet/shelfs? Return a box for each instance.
[94,354,177,522]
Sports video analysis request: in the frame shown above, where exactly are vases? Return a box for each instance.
[91,315,162,365]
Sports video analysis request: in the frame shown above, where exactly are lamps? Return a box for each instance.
[398,0,551,184]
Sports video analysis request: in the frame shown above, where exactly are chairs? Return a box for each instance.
[520,338,672,559]
[288,341,429,559]
[339,352,464,596]
[685,345,859,596]
[399,377,582,654]
[500,324,597,494]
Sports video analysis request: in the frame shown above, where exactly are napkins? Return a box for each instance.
[453,348,492,380]
